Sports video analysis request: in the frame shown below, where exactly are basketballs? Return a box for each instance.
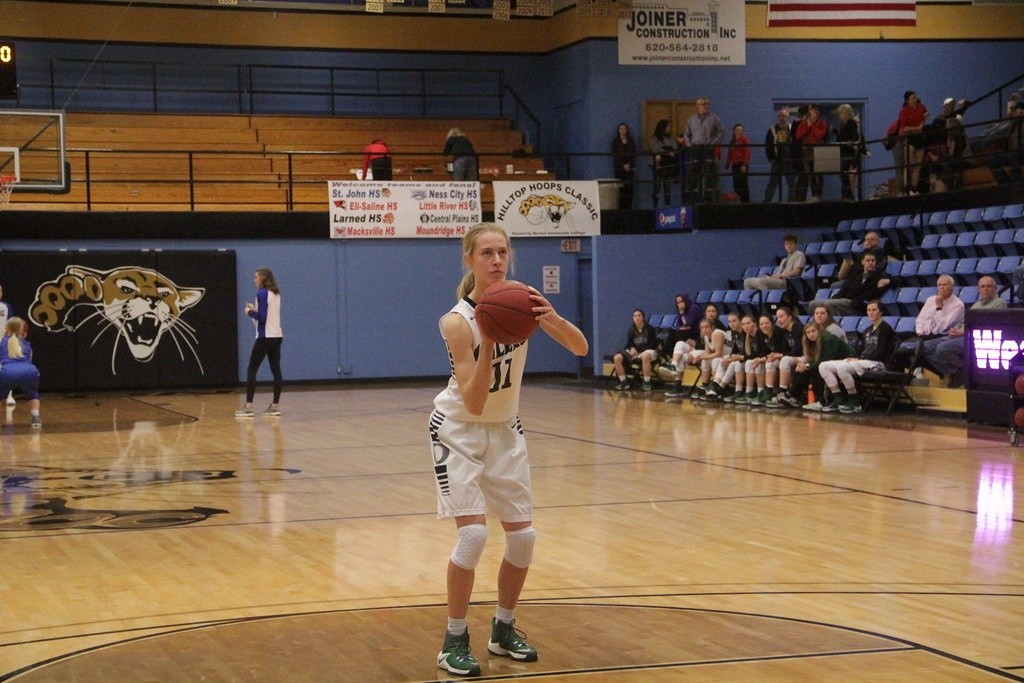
[475,280,543,345]
[22,320,28,337]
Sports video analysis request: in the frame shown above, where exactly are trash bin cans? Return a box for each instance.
[597,179,623,209]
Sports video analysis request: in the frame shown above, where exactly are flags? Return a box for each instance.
[766,0,917,28]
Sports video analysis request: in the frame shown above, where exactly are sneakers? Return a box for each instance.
[437,626,481,676]
[822,400,843,411]
[488,617,538,661]
[838,400,861,413]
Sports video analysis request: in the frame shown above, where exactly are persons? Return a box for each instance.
[0,287,41,426]
[986,86,1024,183]
[744,234,808,291]
[887,91,967,196]
[762,103,871,201]
[444,126,476,180]
[234,268,284,417]
[611,123,637,209]
[650,118,677,209]
[923,276,1007,376]
[809,231,891,315]
[428,222,588,675]
[614,308,657,391]
[722,123,750,203]
[362,139,393,181]
[676,97,726,203]
[915,274,966,337]
[659,292,896,414]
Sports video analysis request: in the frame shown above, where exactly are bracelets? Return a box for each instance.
[936,308,942,311]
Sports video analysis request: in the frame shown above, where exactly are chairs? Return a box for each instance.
[604,203,1022,418]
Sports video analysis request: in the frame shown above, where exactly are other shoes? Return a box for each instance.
[234,404,254,416]
[615,380,630,389]
[664,386,683,397]
[6,397,16,405]
[658,364,678,377]
[802,401,823,411]
[263,404,281,415]
[947,373,964,388]
[640,381,652,391]
[31,416,41,425]
[690,381,799,408]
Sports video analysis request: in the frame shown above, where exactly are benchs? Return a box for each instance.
[0,110,558,214]
